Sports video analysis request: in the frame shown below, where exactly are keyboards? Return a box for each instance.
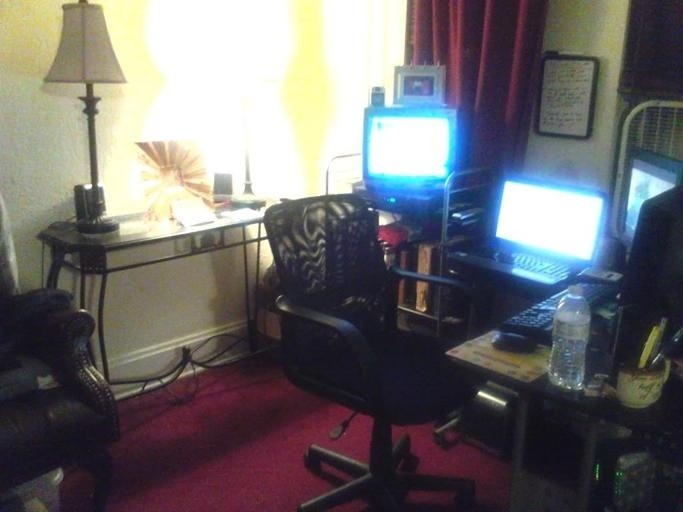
[466,243,574,284]
[500,283,616,336]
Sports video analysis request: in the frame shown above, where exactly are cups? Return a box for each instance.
[613,355,670,410]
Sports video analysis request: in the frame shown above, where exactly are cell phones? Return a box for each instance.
[584,268,623,282]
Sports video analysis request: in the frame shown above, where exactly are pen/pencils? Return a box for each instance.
[637,317,683,371]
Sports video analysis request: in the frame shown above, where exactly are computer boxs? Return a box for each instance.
[352,185,440,215]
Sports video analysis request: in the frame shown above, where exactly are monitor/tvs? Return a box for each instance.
[493,175,609,265]
[616,149,683,242]
[363,105,464,191]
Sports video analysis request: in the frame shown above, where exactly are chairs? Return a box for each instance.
[263,194,491,511]
[0,193,120,512]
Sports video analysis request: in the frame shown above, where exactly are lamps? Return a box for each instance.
[43,0,128,234]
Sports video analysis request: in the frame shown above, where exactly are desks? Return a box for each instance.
[35,206,268,404]
[444,313,683,512]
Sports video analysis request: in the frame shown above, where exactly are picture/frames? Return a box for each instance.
[393,59,446,105]
[533,54,600,140]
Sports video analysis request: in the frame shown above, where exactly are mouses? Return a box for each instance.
[492,332,536,354]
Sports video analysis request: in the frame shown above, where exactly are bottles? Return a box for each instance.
[546,283,592,394]
